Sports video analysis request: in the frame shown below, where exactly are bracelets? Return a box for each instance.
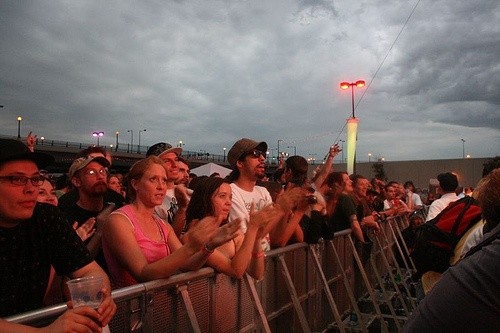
[201,243,215,255]
[252,250,263,258]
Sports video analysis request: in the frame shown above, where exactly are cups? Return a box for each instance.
[66,276,105,333]
[374,212,386,222]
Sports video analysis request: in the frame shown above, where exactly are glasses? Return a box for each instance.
[83,168,108,177]
[245,151,267,158]
[0,174,44,187]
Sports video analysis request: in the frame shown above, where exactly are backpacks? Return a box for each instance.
[411,196,484,273]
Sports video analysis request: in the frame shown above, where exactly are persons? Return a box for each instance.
[0,137,500,333]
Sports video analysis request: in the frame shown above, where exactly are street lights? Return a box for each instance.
[17,116,21,138]
[268,149,277,160]
[368,152,371,162]
[179,140,182,156]
[223,147,226,162]
[461,139,466,159]
[288,146,296,156]
[92,131,104,147]
[340,139,345,163]
[139,129,147,154]
[128,129,133,153]
[340,80,365,173]
[115,130,119,148]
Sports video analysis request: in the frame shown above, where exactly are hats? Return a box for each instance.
[437,172,458,191]
[68,153,111,181]
[146,143,184,158]
[0,139,56,171]
[227,138,268,165]
